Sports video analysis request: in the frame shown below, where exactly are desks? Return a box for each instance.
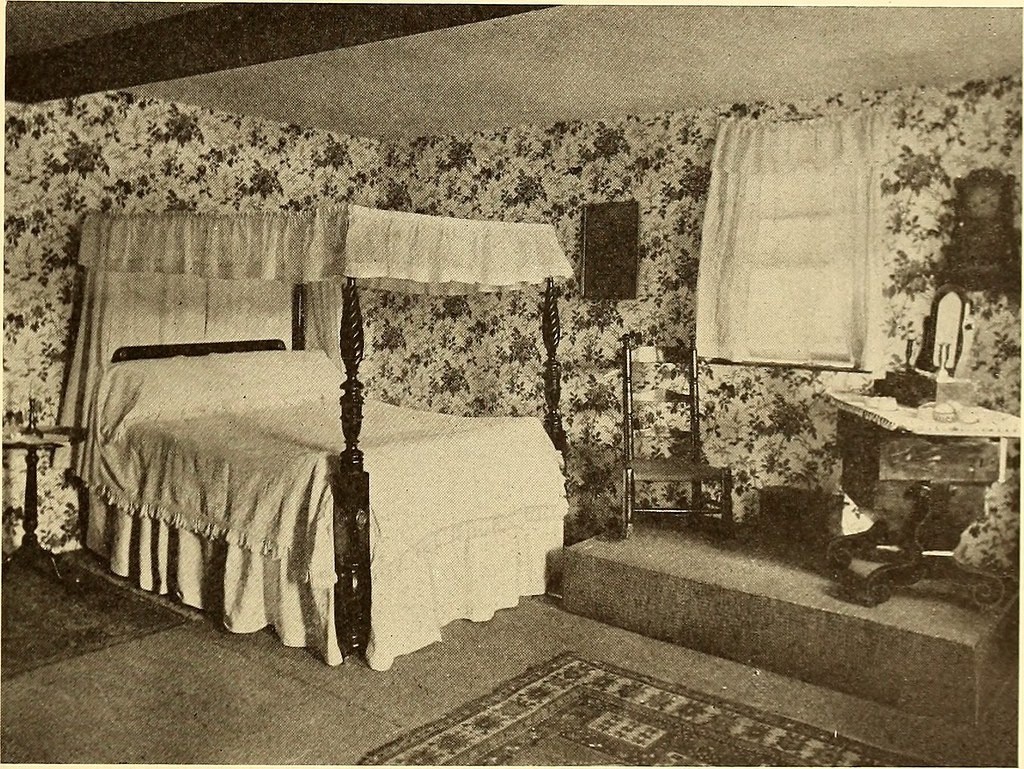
[828,389,1021,538]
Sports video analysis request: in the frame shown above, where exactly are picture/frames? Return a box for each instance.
[580,201,643,298]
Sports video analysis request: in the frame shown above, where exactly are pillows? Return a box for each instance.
[93,350,346,438]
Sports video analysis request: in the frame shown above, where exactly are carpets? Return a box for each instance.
[355,648,948,766]
[2,552,206,683]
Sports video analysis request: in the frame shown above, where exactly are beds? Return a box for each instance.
[55,204,575,672]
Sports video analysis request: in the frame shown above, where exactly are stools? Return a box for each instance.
[1,424,89,593]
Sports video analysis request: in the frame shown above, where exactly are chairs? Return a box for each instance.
[621,331,735,540]
